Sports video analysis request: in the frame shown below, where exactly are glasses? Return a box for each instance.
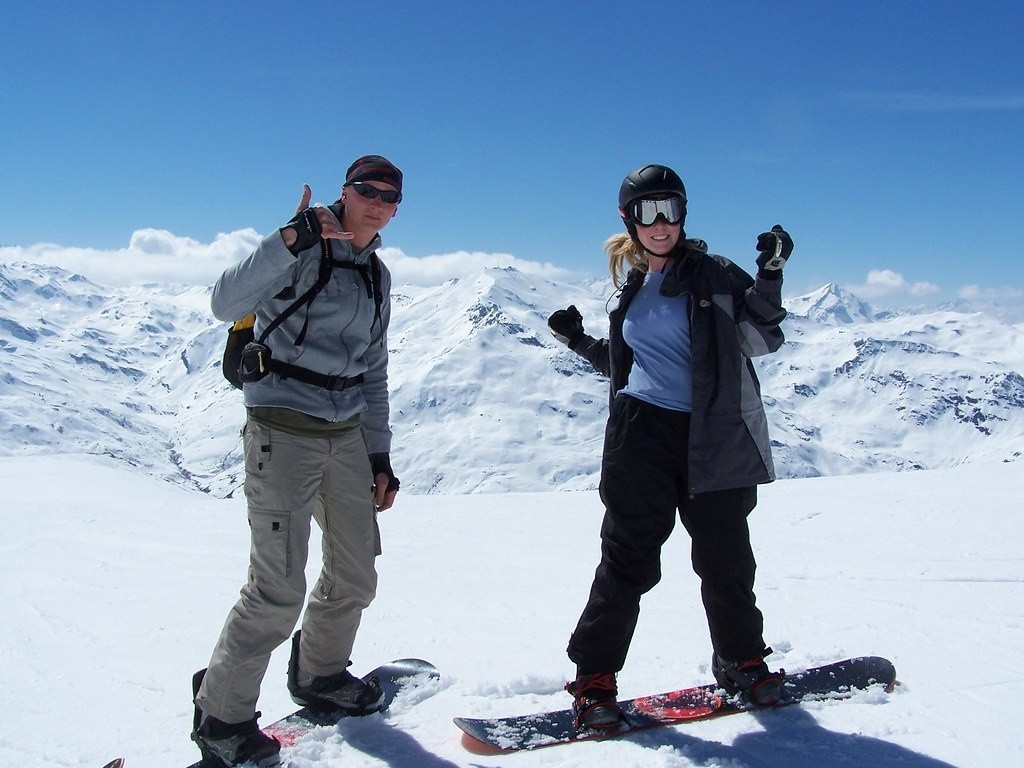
[351,182,401,203]
[627,196,683,226]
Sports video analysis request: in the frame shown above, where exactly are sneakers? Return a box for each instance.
[714,654,781,706]
[196,712,280,768]
[577,665,619,729]
[290,661,385,711]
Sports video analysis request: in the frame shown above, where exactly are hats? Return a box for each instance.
[343,155,403,204]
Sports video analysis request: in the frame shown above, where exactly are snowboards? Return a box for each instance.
[102,657,443,768]
[452,654,897,752]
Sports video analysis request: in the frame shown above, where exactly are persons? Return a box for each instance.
[549,165,794,731]
[194,154,403,768]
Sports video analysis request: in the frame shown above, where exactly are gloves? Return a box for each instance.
[547,305,587,351]
[756,224,794,281]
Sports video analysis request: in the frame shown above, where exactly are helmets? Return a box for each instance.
[618,164,688,234]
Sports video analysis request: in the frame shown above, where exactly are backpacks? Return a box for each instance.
[222,205,384,390]
